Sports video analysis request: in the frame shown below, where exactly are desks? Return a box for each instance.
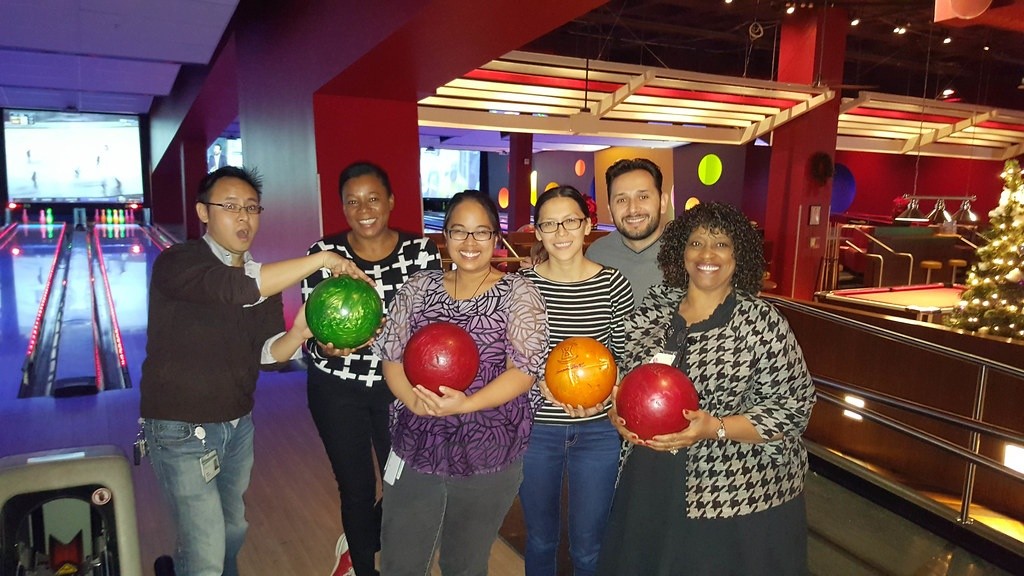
[814,282,975,327]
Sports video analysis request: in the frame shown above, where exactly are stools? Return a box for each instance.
[949,260,967,283]
[921,261,941,285]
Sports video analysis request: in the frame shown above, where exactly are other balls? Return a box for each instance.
[403,322,480,398]
[303,275,382,344]
[546,338,617,410]
[615,363,700,440]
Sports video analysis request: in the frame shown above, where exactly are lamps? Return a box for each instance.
[569,20,600,133]
[895,20,980,223]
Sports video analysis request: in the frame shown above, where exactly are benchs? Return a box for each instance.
[830,213,930,277]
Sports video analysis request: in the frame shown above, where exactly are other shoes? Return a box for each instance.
[331,534,357,576]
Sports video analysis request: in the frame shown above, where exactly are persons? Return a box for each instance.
[208,144,227,173]
[513,157,817,576]
[301,159,442,576]
[371,188,549,576]
[139,164,378,576]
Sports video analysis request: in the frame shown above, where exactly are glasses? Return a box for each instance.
[196,201,265,214]
[445,228,497,241]
[534,217,587,233]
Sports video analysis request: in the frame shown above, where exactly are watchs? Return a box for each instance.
[715,415,726,440]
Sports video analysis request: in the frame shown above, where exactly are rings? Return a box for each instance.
[669,447,679,455]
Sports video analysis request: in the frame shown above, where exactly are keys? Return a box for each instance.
[133,427,149,466]
